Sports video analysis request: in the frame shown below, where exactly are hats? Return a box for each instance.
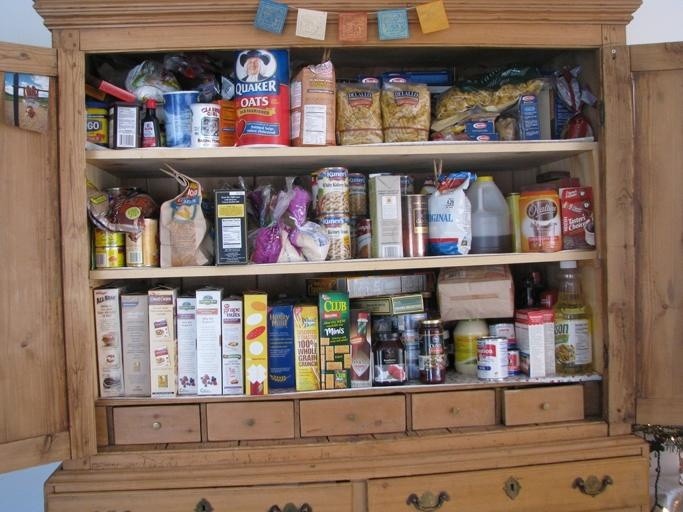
[240,50,270,67]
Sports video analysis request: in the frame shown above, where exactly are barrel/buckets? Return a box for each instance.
[466,176,510,253]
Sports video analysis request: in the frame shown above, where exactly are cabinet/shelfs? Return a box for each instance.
[0,0,682,512]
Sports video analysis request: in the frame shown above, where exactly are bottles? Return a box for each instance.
[138,99,162,149]
[553,261,595,378]
[348,311,371,382]
[421,291,434,320]
[517,270,545,308]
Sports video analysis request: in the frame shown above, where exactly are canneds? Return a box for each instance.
[401,193,429,257]
[94,219,158,269]
[399,175,415,196]
[311,167,372,261]
[372,312,446,386]
[476,323,521,381]
[190,102,221,148]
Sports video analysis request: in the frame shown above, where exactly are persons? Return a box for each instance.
[239,50,271,83]
[22,84,40,119]
